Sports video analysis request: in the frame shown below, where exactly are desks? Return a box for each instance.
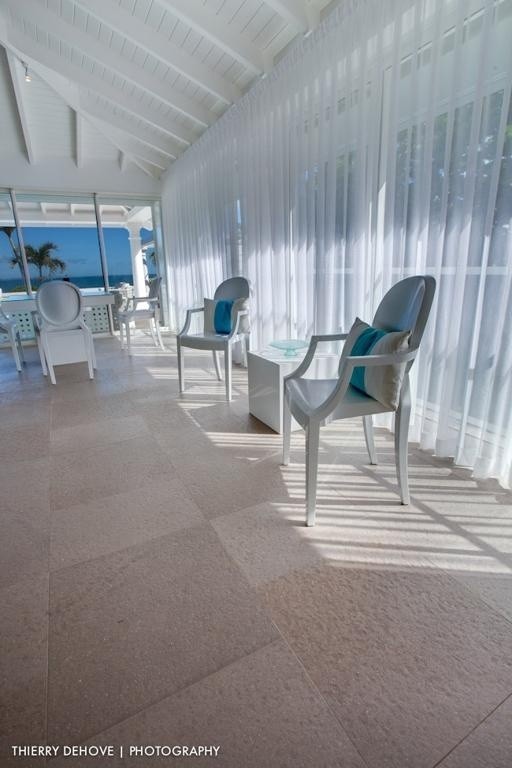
[1,291,116,314]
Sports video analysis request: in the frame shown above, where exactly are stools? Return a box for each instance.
[0,306,26,372]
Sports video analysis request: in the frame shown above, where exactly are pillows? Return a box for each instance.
[203,296,251,338]
[337,316,411,411]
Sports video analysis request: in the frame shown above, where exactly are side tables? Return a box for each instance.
[246,349,337,437]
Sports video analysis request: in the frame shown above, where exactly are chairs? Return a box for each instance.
[281,274,437,525]
[175,276,253,404]
[30,279,97,384]
[117,277,165,356]
